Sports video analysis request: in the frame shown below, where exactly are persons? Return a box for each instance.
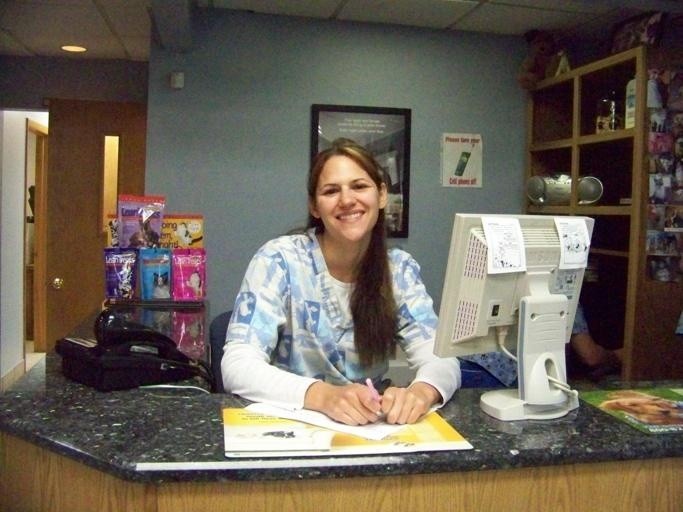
[451,282,625,386]
[217,135,462,429]
[644,62,670,107]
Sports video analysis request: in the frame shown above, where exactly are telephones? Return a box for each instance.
[53,309,204,394]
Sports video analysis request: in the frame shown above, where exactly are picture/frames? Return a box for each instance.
[311,104,412,239]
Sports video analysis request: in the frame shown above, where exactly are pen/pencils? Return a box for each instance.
[365,377,385,417]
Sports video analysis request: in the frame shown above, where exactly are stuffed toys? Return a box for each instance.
[552,47,577,81]
[514,28,551,94]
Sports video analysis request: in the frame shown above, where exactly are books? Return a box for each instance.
[215,407,475,457]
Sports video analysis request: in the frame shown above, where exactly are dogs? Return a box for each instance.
[597,390,682,427]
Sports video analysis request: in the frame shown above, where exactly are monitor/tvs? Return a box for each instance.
[431,212,596,421]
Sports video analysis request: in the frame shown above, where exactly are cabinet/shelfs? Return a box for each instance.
[522,47,683,382]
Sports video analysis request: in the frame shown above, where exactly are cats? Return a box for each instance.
[651,177,667,204]
